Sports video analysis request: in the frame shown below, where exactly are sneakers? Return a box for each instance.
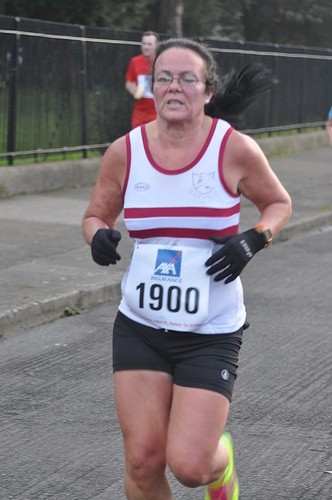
[204,431,240,499]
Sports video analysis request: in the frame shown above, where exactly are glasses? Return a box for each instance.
[153,74,208,88]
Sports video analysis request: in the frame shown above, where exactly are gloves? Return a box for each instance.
[204,227,268,285]
[91,228,122,268]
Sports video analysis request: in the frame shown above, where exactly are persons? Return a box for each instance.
[325,105,332,142]
[125,31,161,128]
[82,38,293,499]
